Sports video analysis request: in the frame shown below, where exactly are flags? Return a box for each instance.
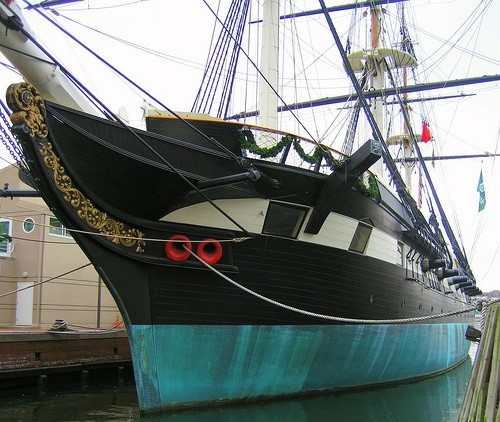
[476,168,486,213]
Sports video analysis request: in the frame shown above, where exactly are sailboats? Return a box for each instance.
[0,0,500,418]
